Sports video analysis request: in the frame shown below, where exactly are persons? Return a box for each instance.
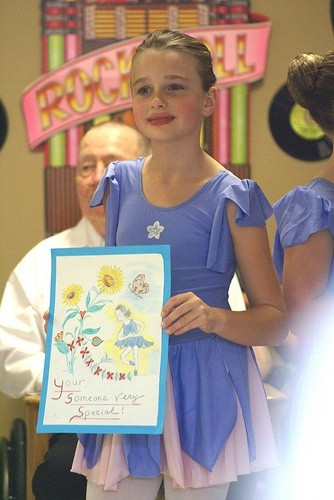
[0,120,152,500]
[43,32,289,500]
[272,52,334,354]
